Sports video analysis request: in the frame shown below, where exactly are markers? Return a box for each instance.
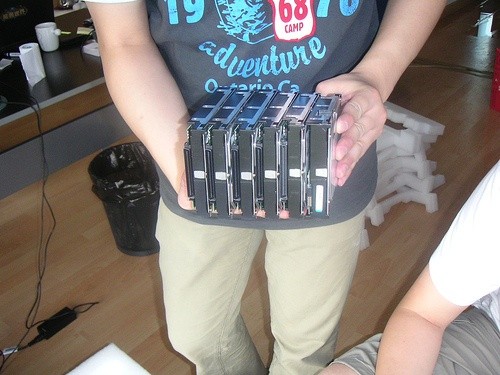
[3,53,20,56]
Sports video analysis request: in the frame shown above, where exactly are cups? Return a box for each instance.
[35,22,59,52]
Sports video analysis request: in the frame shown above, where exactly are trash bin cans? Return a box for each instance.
[88,142,161,256]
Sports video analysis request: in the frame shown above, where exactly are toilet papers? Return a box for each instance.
[18,42,47,87]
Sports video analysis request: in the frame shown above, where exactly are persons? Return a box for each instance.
[314,158,500,375]
[85,0,448,375]
